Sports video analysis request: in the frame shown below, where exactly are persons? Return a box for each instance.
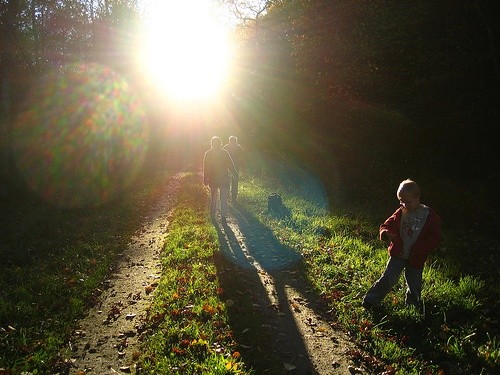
[222,136,246,204]
[360,178,443,311]
[202,136,240,225]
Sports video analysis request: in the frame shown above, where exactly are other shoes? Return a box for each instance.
[361,299,371,306]
[408,298,421,307]
